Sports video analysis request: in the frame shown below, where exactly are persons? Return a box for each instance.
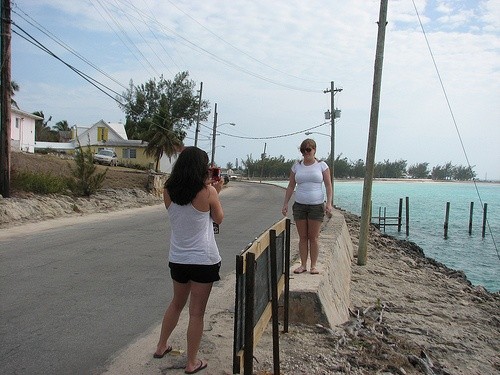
[281,139,333,274]
[153,147,222,374]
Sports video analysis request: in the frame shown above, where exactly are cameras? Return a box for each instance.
[211,169,220,183]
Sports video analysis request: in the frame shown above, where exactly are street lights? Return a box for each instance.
[211,122,237,169]
[303,131,334,207]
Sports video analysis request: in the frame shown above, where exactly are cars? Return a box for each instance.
[91,150,118,166]
[220,173,238,184]
